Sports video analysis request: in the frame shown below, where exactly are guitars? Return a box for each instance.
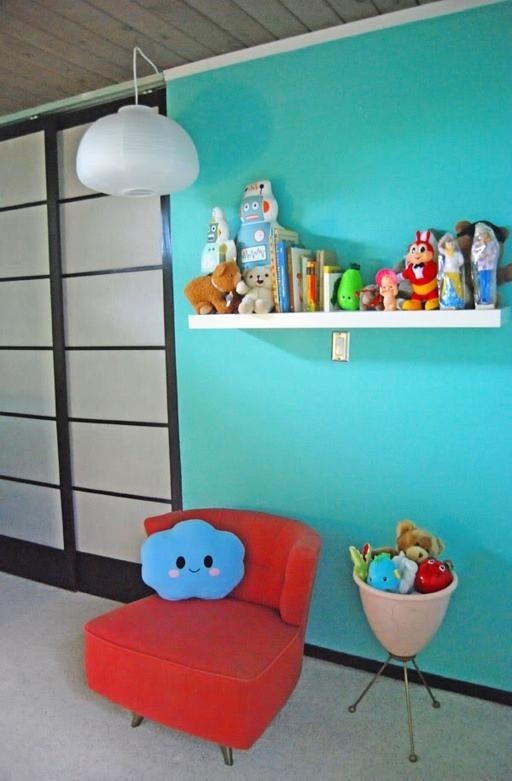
[73,104,201,202]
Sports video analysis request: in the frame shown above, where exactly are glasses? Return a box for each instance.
[75,47,199,203]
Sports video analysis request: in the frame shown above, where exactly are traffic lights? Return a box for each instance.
[139,520,247,603]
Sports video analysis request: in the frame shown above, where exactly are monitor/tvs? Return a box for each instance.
[268,225,344,313]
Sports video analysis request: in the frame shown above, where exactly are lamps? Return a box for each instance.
[80,504,323,770]
